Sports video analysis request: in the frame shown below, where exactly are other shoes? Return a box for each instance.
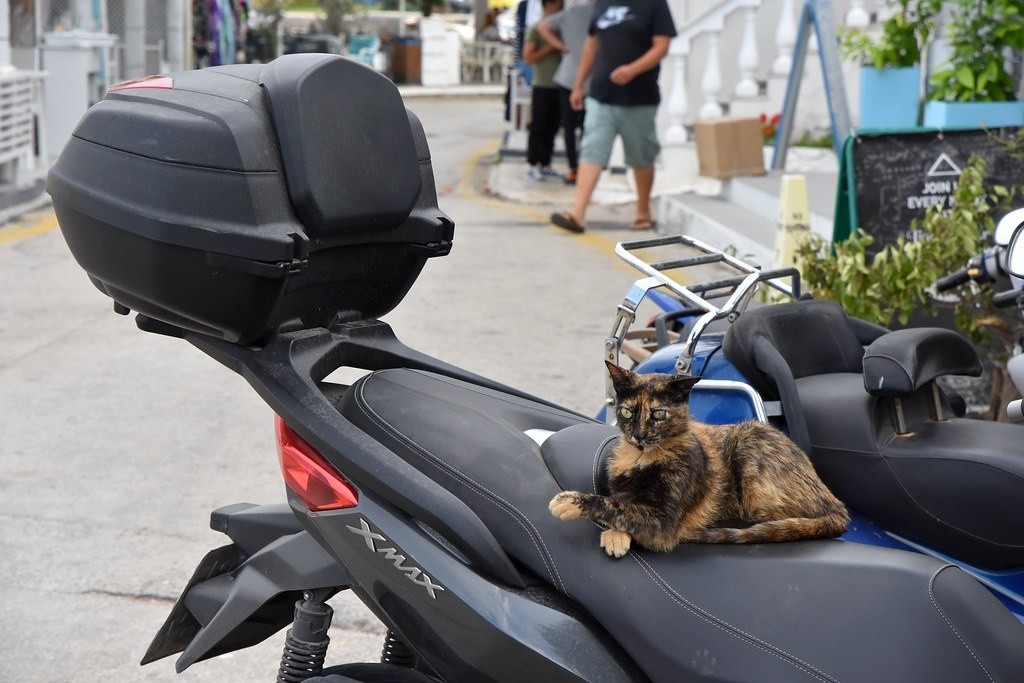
[565,172,578,183]
[528,164,545,181]
[541,165,561,178]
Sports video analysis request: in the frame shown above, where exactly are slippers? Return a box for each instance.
[629,219,655,229]
[551,212,585,234]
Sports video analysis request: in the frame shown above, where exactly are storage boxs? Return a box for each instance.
[694,118,768,176]
[47,54,452,338]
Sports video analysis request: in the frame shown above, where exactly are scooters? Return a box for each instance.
[592,210,1023,620]
[44,52,1024,683]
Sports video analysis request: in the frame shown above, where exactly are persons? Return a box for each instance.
[505,0,594,182]
[550,0,678,233]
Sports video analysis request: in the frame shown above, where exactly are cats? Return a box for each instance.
[548,360,851,559]
